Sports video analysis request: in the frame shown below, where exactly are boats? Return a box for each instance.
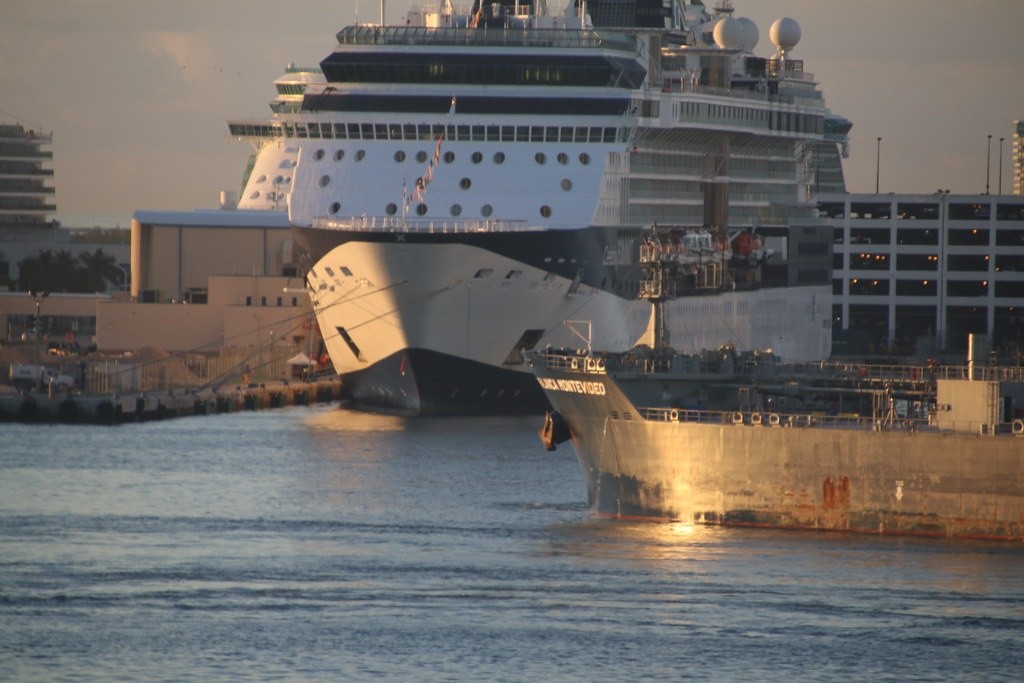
[520,328,1024,549]
[220,1,855,231]
[302,219,842,417]
